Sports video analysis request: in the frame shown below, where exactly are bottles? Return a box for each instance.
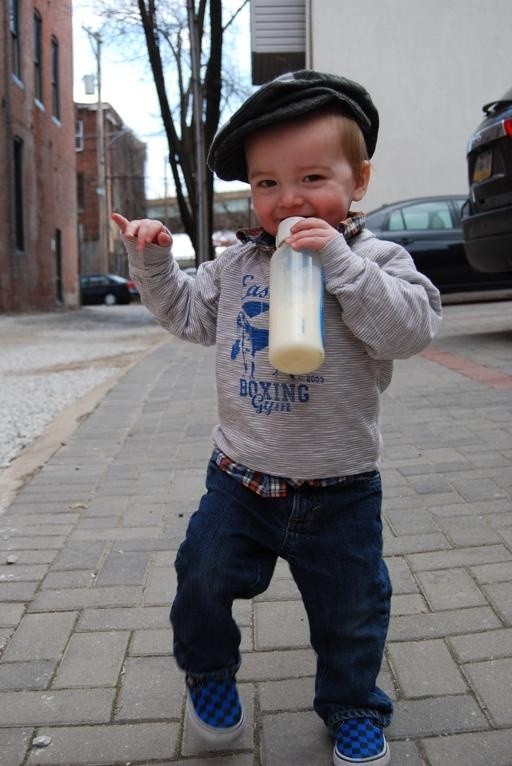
[269,217,325,375]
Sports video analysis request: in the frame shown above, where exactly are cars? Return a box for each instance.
[362,81,512,303]
[80,273,136,305]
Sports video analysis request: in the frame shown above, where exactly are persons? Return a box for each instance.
[110,67,442,766]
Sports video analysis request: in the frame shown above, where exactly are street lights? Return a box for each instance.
[75,26,109,272]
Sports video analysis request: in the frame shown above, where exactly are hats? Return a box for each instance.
[207,70,379,184]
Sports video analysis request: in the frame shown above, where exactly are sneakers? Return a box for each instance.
[332,715,390,765]
[186,674,246,744]
